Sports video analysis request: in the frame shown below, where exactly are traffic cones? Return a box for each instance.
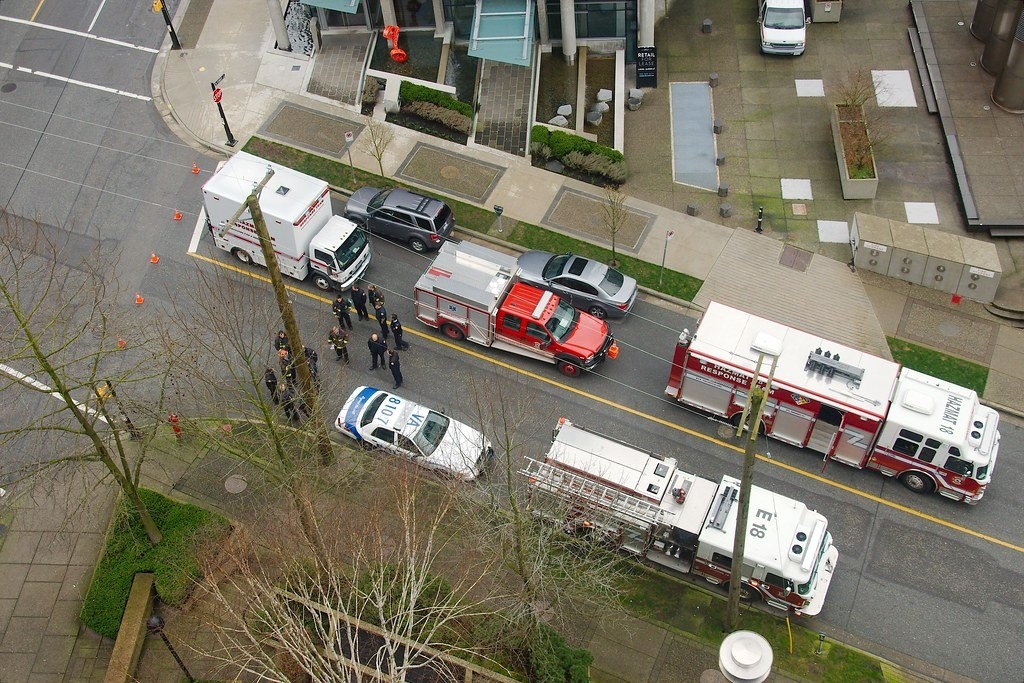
[174,208,182,220]
[191,162,200,174]
[609,340,619,359]
[117,335,126,347]
[135,292,144,303]
[150,251,160,263]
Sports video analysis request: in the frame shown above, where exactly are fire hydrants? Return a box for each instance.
[168,412,181,438]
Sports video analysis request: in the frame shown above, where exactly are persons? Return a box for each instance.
[279,383,300,422]
[302,346,318,362]
[375,302,389,340]
[386,349,402,389]
[367,334,388,370]
[351,284,369,321]
[306,357,320,395]
[332,295,353,330]
[275,331,292,355]
[279,351,298,394]
[390,314,409,351]
[266,368,279,404]
[327,326,350,364]
[368,284,385,309]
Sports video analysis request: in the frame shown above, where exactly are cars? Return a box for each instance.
[517,249,639,321]
[334,385,494,483]
[757,0,810,57]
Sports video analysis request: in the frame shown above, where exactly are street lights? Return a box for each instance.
[147,615,194,683]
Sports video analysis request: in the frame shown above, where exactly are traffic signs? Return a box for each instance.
[214,74,225,87]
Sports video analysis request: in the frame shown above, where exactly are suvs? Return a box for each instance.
[343,187,456,254]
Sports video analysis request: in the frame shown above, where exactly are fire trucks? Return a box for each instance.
[516,416,840,619]
[664,299,1000,505]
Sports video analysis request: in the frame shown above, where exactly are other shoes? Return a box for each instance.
[335,355,342,361]
[394,346,402,349]
[382,364,386,369]
[393,383,401,388]
[349,325,353,330]
[345,358,349,365]
[370,365,377,370]
[403,343,409,350]
[359,314,363,321]
[364,315,369,320]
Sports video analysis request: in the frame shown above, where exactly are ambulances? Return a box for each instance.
[201,150,371,292]
[413,240,613,377]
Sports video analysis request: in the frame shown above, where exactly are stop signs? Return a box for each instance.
[213,89,223,103]
